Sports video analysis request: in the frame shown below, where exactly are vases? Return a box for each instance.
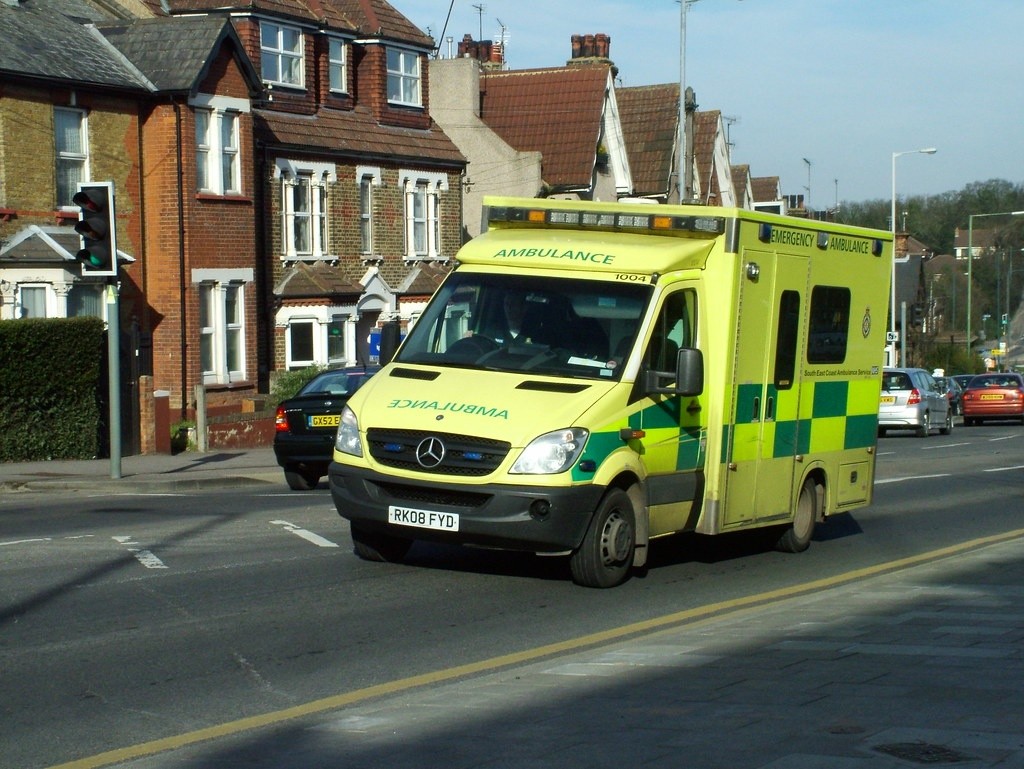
[596,154,610,164]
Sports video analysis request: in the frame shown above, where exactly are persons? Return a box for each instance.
[483,289,539,348]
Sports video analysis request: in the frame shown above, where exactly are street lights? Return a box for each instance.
[892,148,936,364]
[967,211,1024,373]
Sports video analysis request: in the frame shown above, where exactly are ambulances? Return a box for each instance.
[326,196,892,592]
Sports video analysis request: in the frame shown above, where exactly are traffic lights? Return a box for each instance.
[1002,314,1006,325]
[71,181,117,278]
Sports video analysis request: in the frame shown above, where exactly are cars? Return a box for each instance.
[875,368,951,437]
[273,367,392,490]
[961,373,1024,426]
[932,375,975,416]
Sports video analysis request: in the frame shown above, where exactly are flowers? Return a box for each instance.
[598,144,607,154]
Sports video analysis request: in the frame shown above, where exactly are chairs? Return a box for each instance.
[488,292,553,345]
[976,378,990,388]
[612,314,678,391]
